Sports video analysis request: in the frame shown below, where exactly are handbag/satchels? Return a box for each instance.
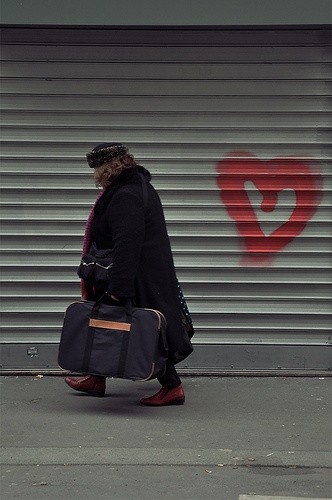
[77,254,112,283]
[57,275,168,382]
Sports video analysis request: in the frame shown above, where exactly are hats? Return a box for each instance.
[86,142,129,169]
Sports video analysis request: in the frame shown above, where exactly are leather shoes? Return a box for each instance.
[141,382,185,407]
[64,373,106,398]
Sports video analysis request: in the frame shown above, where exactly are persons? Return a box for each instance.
[56,144,196,406]
